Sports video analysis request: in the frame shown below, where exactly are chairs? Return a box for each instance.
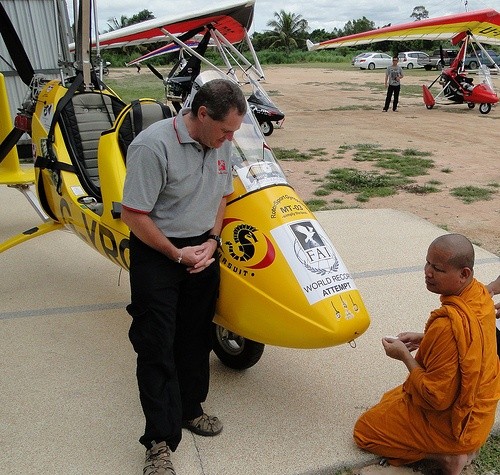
[123,101,171,168]
[60,92,123,195]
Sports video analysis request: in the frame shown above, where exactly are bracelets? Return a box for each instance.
[208,235,223,249]
[175,249,183,264]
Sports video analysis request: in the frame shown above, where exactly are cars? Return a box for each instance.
[354,53,393,70]
[398,52,430,69]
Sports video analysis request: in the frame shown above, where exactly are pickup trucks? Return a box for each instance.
[416,49,481,71]
[449,50,500,68]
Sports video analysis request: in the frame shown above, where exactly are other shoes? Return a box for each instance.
[143,442,176,475]
[189,414,222,436]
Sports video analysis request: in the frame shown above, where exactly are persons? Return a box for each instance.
[482,274,500,359]
[119,78,247,475]
[352,232,500,475]
[382,55,404,112]
